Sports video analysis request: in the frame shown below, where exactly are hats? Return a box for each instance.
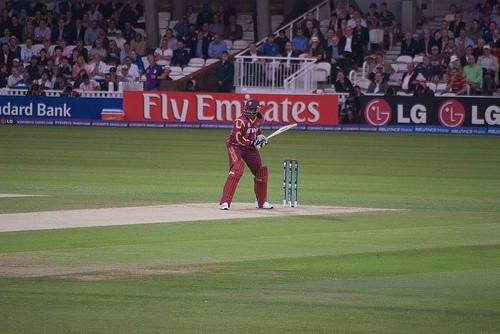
[483,45,490,49]
[110,67,116,71]
[450,55,458,62]
[13,58,19,62]
[9,36,16,40]
[432,46,439,50]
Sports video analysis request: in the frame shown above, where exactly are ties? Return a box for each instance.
[312,37,319,42]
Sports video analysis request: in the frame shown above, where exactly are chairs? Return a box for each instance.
[316,29,446,95]
[18,10,283,80]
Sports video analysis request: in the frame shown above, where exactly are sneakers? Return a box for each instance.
[255,201,273,209]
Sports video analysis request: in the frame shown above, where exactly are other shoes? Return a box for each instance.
[220,203,228,210]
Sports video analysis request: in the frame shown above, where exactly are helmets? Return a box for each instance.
[245,99,258,109]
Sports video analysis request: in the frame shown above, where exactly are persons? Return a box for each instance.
[0,0,500,126]
[218,99,273,210]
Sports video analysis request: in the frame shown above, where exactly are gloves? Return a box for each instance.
[253,134,268,149]
